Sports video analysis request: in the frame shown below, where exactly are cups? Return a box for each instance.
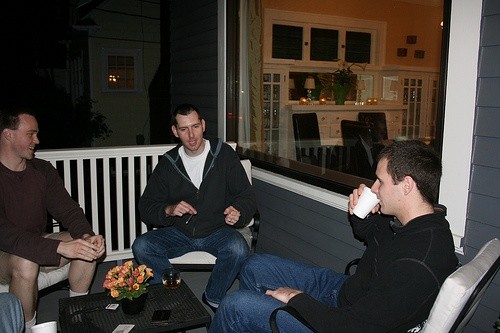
[352,186,380,219]
[161,267,182,289]
[300,97,307,104]
[367,98,378,105]
[319,98,326,105]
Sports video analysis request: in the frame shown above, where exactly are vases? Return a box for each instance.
[118,294,148,314]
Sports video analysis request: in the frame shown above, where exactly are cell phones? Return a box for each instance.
[151,309,172,324]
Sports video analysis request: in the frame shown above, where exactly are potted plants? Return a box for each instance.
[322,63,357,105]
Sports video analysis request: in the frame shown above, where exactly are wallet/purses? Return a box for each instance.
[151,309,172,324]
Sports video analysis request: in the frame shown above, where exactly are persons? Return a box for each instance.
[209,138,460,333]
[131,103,257,312]
[0,103,105,333]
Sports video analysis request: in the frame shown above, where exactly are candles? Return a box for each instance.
[320,98,327,105]
[300,97,307,105]
[366,98,378,105]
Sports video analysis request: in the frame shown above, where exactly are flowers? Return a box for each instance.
[104,262,153,301]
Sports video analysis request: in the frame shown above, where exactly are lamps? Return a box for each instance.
[305,76,316,104]
[358,80,365,103]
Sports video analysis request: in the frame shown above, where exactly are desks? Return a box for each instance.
[60,279,211,333]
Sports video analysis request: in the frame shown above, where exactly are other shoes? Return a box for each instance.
[202,292,219,315]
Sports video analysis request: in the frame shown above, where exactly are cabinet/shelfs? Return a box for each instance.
[278,102,408,161]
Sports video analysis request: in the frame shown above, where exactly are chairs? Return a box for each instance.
[167,160,259,279]
[292,112,388,181]
[0,222,72,294]
[270,239,500,333]
